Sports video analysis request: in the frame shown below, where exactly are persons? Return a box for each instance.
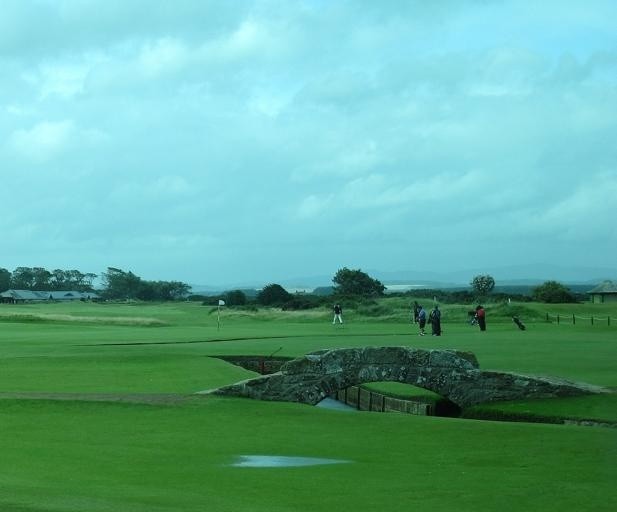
[415,305,426,337]
[430,305,440,337]
[331,303,343,324]
[471,305,483,326]
[412,300,420,325]
[475,306,486,332]
